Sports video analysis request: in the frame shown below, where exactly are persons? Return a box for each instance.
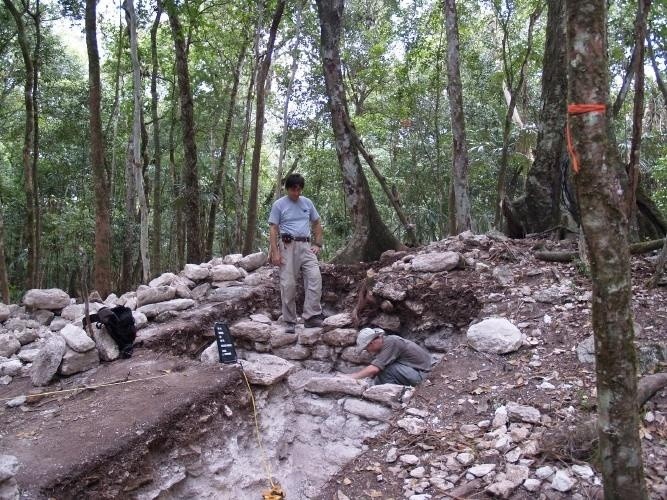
[335,326,434,388]
[268,172,327,334]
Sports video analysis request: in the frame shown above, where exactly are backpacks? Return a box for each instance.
[99,304,136,347]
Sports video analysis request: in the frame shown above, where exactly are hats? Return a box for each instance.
[356,328,379,355]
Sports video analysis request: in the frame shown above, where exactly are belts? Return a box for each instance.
[294,237,310,242]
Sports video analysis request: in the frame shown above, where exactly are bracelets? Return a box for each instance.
[315,241,323,249]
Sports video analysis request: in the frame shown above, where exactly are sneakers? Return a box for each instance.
[304,315,323,328]
[285,323,295,333]
